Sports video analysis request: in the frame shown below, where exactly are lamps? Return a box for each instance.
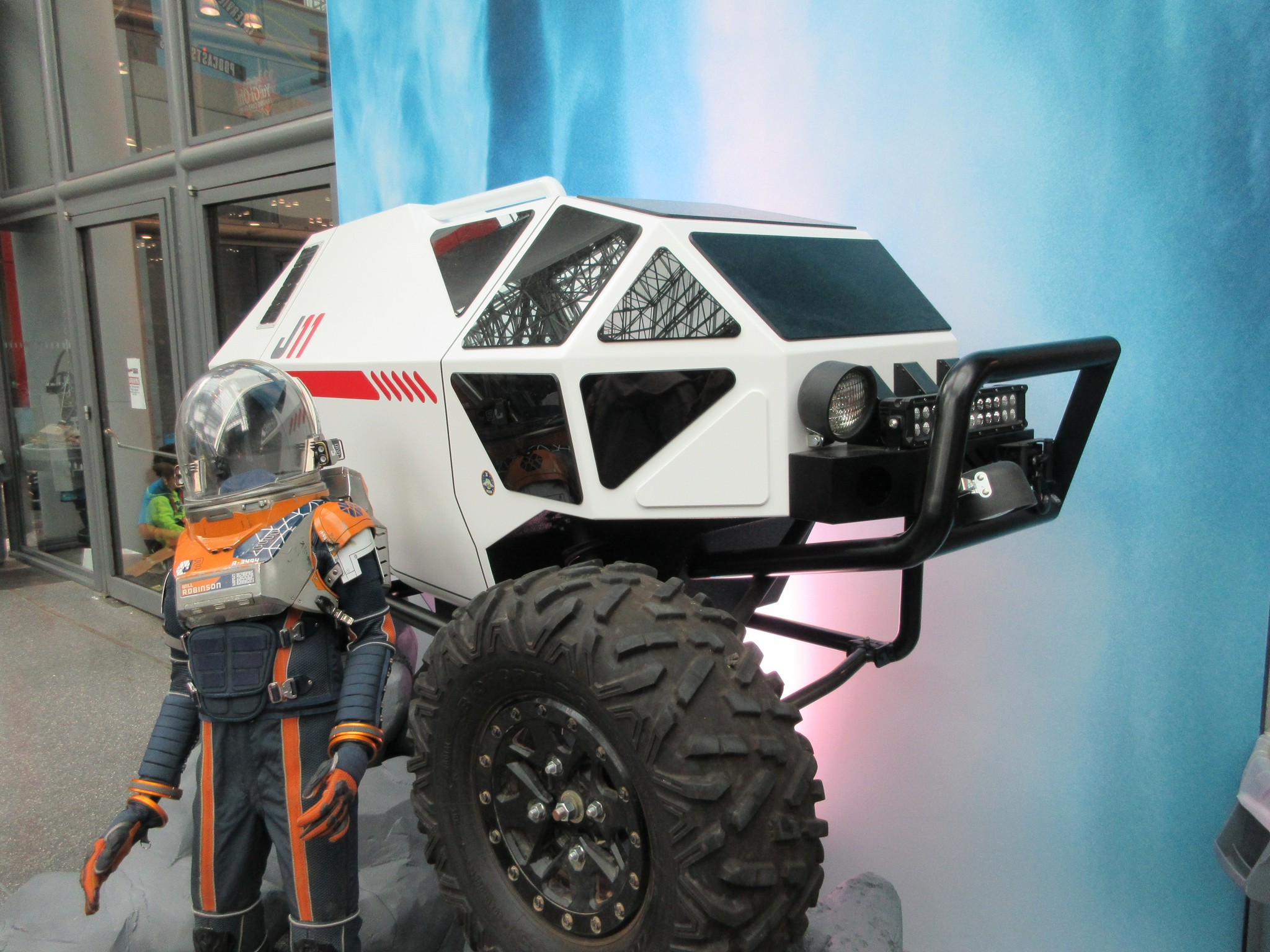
[242,0,263,30]
[228,198,336,233]
[199,0,222,18]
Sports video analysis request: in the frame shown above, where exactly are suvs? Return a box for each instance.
[202,172,1125,952]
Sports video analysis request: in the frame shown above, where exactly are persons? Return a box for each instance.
[134,444,186,571]
[79,360,399,951]
